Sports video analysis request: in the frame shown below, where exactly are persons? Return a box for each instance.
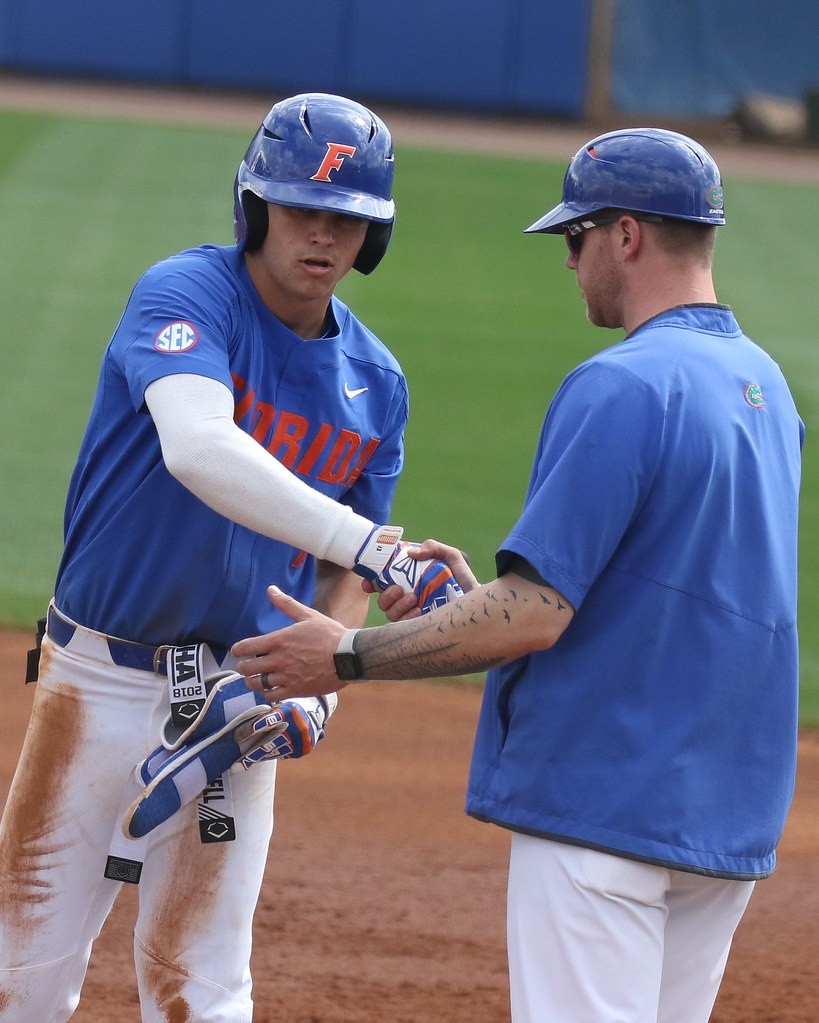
[229,128,808,1023]
[0,94,554,1023]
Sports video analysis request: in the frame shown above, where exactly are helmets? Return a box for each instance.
[232,92,396,276]
[523,128,726,236]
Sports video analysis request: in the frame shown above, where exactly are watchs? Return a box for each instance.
[333,628,373,680]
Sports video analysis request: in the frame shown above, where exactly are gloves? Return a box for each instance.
[349,522,465,616]
[227,686,338,776]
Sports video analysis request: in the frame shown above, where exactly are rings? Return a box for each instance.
[261,673,271,690]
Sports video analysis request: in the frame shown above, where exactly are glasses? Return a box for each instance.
[561,217,665,258]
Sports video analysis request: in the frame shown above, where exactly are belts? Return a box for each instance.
[47,604,229,681]
